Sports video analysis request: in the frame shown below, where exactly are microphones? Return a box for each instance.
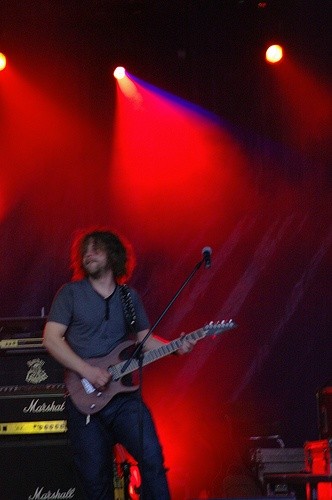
[202,246,212,267]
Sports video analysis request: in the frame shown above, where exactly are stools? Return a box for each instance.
[263,474,332,500]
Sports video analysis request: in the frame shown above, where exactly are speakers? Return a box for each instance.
[0,394,83,500]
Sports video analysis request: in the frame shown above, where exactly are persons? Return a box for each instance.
[42,227,196,500]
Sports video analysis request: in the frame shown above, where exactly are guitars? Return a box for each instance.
[64,319,236,415]
